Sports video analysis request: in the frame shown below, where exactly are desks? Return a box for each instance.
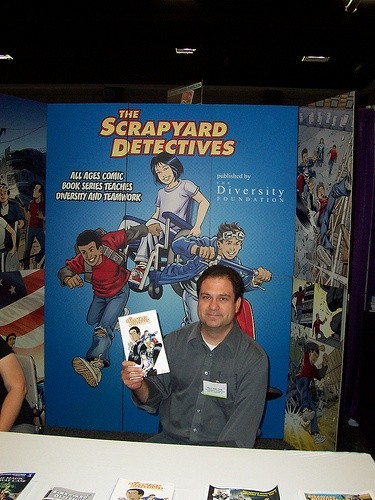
[0,431,375,500]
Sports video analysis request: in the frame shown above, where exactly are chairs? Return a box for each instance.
[14,354,42,432]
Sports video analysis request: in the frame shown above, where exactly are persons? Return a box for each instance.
[0,336,37,434]
[122,265,269,448]
